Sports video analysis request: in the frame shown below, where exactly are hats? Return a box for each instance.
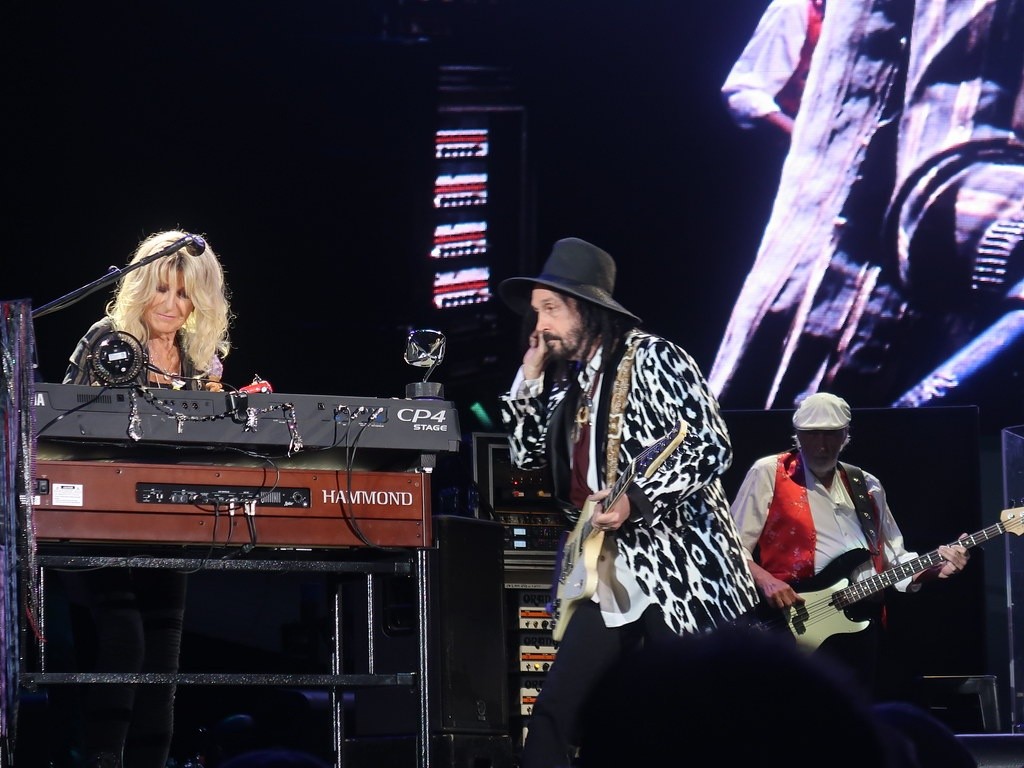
[497,236,641,326]
[792,393,851,430]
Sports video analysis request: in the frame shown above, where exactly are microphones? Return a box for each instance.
[185,235,206,258]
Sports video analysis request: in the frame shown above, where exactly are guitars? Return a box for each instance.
[750,508,1024,667]
[551,418,690,641]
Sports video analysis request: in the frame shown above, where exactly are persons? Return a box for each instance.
[572,616,978,767]
[497,235,763,768]
[729,392,971,768]
[60,229,234,398]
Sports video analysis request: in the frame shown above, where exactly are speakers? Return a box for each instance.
[343,515,505,768]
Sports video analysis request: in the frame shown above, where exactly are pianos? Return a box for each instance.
[0,382,462,767]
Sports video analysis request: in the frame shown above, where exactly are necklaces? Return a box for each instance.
[144,336,181,381]
